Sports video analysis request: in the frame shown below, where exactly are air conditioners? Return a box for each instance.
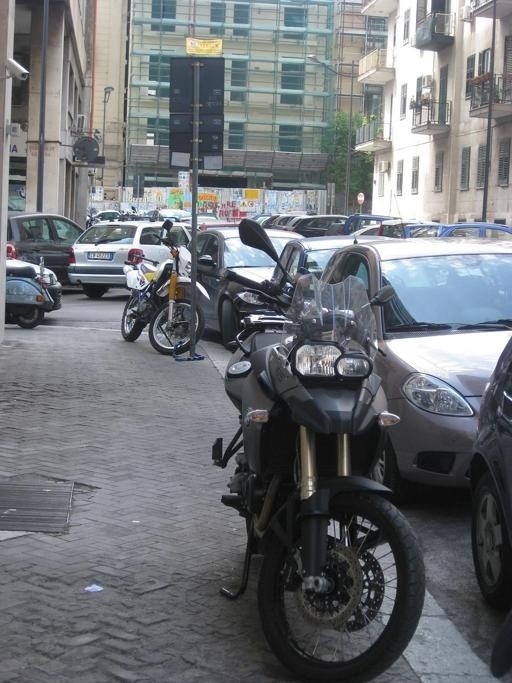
[458,5,475,25]
[378,159,387,173]
[423,75,431,89]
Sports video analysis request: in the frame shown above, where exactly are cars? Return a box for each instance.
[464,332,512,624]
[311,234,512,503]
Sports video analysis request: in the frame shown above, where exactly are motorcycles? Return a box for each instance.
[204,217,431,682]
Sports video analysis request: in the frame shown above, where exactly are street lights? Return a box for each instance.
[99,85,117,188]
